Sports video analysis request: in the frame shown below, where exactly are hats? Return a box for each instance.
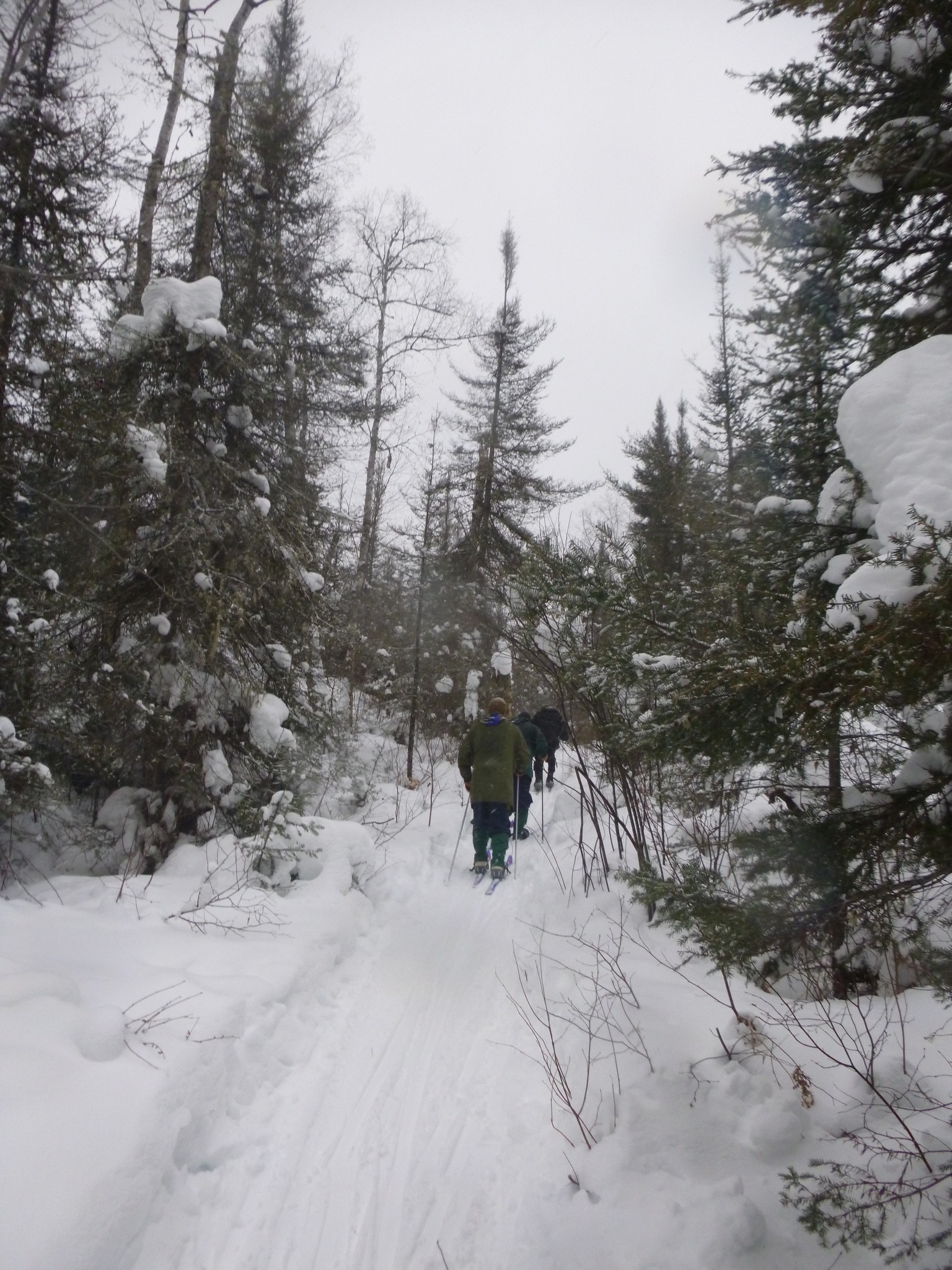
[519,711,531,722]
[488,698,507,714]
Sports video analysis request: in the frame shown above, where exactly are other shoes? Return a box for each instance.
[546,778,554,789]
[490,865,504,879]
[520,828,529,839]
[474,861,487,874]
[535,778,543,790]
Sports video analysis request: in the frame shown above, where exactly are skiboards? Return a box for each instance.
[489,820,514,849]
[536,787,551,794]
[472,850,513,895]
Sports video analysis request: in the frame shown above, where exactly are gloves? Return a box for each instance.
[514,769,523,776]
[542,753,548,761]
[465,779,472,791]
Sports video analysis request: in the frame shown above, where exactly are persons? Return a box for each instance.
[457,697,529,880]
[509,712,548,840]
[531,704,571,791]
